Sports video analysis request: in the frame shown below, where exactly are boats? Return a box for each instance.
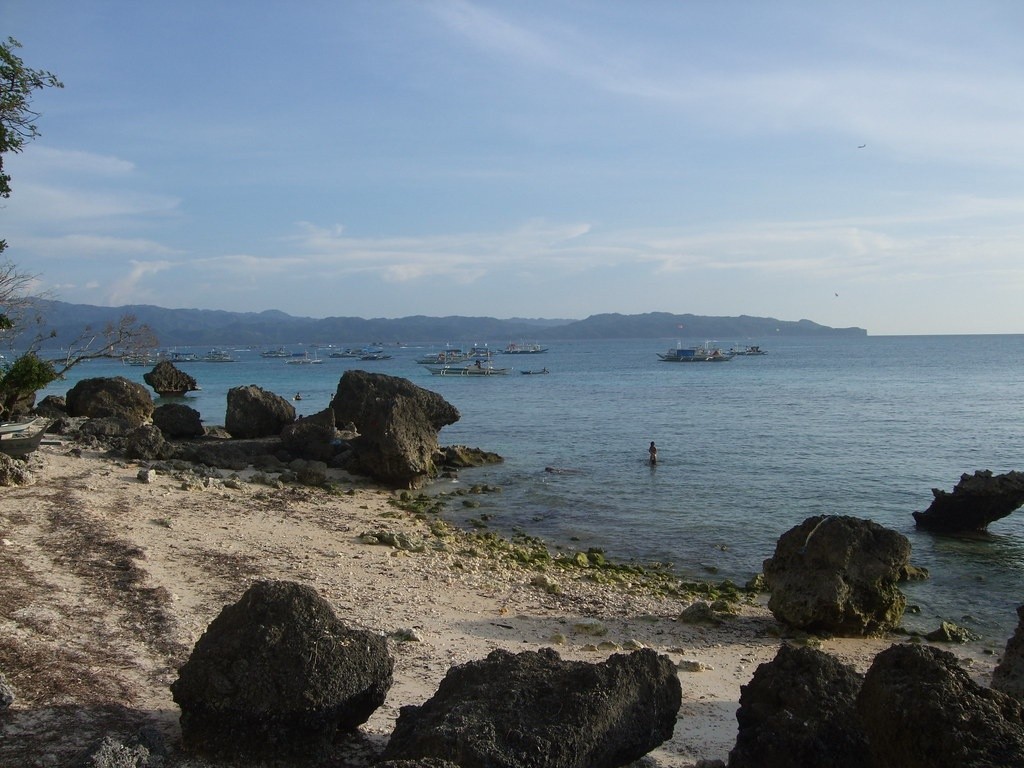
[654,340,737,361]
[521,366,550,374]
[737,345,768,356]
[126,337,550,376]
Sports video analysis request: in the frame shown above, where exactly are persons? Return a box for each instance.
[649,441,657,465]
[296,414,303,421]
[296,392,301,400]
[331,393,334,401]
